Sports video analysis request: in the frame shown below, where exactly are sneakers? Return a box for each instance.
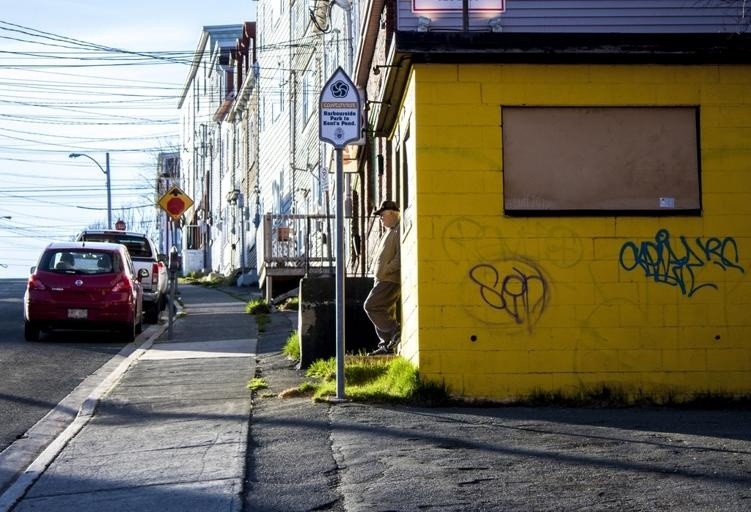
[367,346,395,356]
[387,325,400,349]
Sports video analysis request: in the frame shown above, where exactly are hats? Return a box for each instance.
[373,201,399,215]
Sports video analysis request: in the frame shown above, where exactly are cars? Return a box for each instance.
[22,239,149,345]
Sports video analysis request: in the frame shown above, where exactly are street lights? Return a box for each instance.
[0,216,12,220]
[69,149,111,229]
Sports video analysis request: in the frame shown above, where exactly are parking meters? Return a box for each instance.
[167,244,180,340]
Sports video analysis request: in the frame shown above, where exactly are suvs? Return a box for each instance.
[76,229,169,327]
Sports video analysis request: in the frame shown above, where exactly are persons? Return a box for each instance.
[361,202,401,355]
